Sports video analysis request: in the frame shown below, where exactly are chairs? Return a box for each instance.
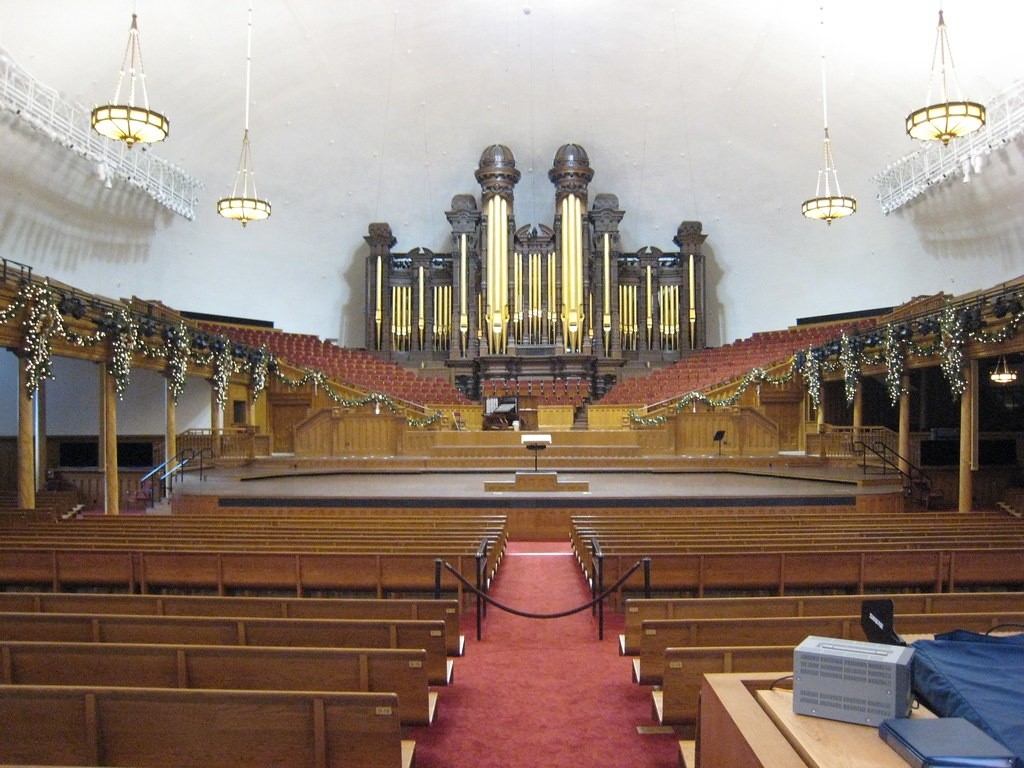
[479,377,592,414]
[197,322,472,406]
[453,412,468,430]
[597,318,878,407]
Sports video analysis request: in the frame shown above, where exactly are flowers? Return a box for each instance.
[95,301,148,401]
[243,341,291,403]
[799,344,835,411]
[293,361,441,430]
[937,296,1024,399]
[149,319,206,406]
[834,333,875,408]
[0,276,95,401]
[206,334,241,411]
[875,323,936,407]
[627,355,797,425]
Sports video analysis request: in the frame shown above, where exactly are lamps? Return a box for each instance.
[990,354,1018,383]
[906,4,986,148]
[88,16,168,154]
[992,293,1024,340]
[217,0,271,230]
[971,295,988,326]
[800,4,855,226]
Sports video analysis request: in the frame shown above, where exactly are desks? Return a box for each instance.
[691,633,1016,767]
[521,435,551,471]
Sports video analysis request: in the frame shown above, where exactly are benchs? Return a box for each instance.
[481,414,510,431]
[566,512,1024,725]
[0,508,511,768]
